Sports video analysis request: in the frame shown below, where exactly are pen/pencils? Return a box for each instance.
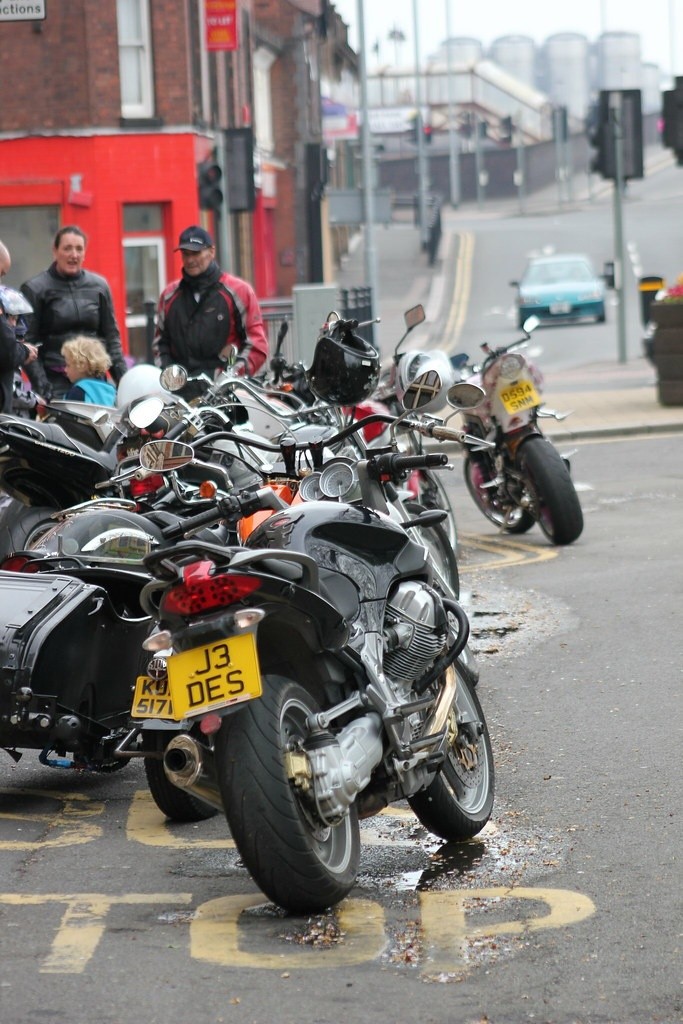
[34,342,44,347]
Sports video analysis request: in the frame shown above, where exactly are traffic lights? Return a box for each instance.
[196,161,227,213]
[587,89,618,182]
[409,115,418,146]
[655,75,683,164]
[500,115,514,145]
[425,125,432,144]
[479,122,489,140]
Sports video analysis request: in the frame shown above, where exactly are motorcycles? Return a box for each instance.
[1,312,519,911]
[446,315,585,547]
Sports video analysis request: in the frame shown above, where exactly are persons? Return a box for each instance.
[1,225,127,416]
[153,226,268,404]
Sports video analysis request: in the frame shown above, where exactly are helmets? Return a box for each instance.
[395,349,453,412]
[309,330,380,403]
[118,364,163,414]
[0,285,34,335]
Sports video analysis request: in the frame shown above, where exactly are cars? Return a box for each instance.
[513,253,606,329]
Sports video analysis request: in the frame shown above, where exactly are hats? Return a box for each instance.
[172,226,212,252]
